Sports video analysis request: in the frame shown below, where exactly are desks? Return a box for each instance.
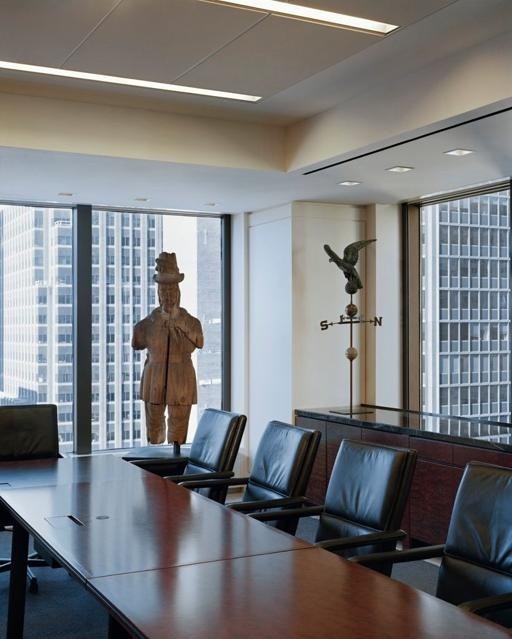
[1,455,511,638]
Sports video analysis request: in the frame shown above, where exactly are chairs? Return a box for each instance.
[0,404,64,594]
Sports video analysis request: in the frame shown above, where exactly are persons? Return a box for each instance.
[131,251,204,444]
